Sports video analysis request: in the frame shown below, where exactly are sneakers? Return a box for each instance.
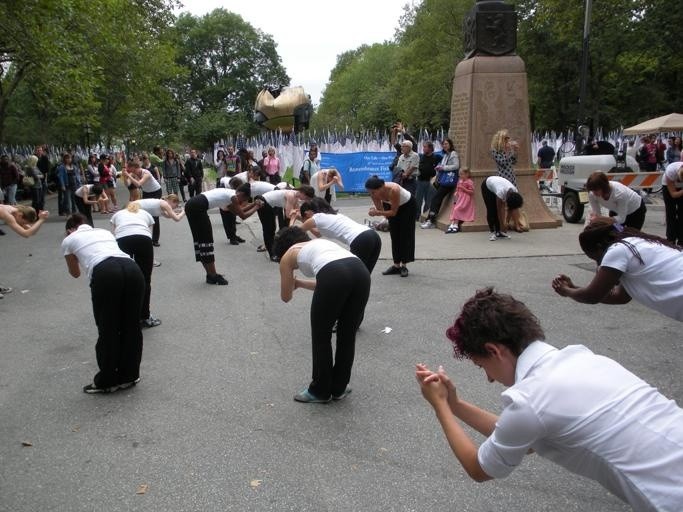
[295,389,332,404]
[1,286,12,294]
[382,220,523,277]
[84,382,117,394]
[206,274,228,285]
[140,316,160,327]
[333,384,352,400]
[116,378,140,389]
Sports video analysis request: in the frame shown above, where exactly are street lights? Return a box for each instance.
[131,138,136,152]
[82,120,93,158]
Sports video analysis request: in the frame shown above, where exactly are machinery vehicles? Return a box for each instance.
[541,0,665,224]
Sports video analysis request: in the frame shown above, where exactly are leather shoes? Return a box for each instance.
[236,236,245,242]
[153,242,160,246]
[230,238,239,245]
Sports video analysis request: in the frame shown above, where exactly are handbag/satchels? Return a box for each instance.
[437,169,458,188]
[22,175,36,186]
[299,166,311,185]
[509,209,530,231]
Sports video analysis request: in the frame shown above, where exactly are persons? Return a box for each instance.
[185,183,263,285]
[366,175,418,277]
[56,151,126,227]
[291,198,381,331]
[269,225,371,402]
[111,193,185,327]
[414,286,682,512]
[537,135,683,321]
[0,146,52,214]
[216,144,321,185]
[1,204,50,297]
[62,213,147,393]
[220,166,344,262]
[128,147,204,202]
[389,131,523,241]
